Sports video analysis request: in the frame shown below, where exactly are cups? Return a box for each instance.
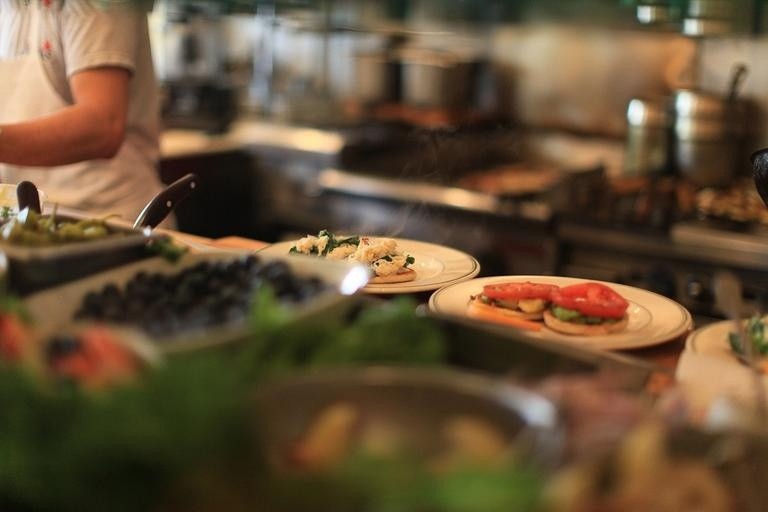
[626,87,754,185]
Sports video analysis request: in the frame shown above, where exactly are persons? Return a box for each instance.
[1,0,182,232]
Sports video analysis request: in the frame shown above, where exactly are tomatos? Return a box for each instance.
[484,282,628,319]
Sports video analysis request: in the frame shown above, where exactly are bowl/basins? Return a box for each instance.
[249,368,562,480]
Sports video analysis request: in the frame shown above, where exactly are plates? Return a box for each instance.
[251,234,481,295]
[23,253,367,360]
[427,276,693,351]
[674,319,768,409]
